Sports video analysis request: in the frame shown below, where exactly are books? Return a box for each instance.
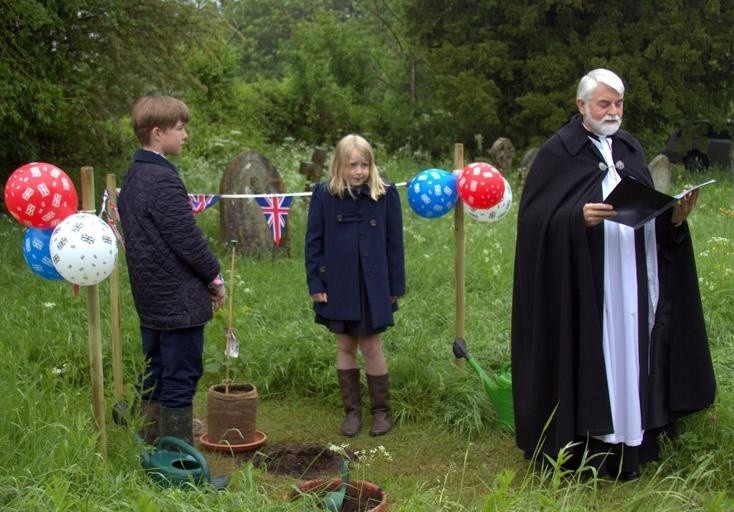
[601,179,716,229]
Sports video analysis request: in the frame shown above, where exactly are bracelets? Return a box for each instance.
[208,277,225,293]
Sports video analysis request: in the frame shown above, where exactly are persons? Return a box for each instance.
[305,135,407,440]
[115,96,228,456]
[511,70,718,482]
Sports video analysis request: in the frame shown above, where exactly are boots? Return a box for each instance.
[138,400,162,446]
[337,366,364,438]
[365,371,395,437]
[157,404,231,492]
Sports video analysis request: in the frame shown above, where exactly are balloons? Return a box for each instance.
[50,213,118,287]
[3,161,78,231]
[458,161,505,209]
[408,168,457,219]
[21,227,64,282]
[463,180,514,224]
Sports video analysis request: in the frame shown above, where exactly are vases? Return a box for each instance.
[284,473,387,512]
